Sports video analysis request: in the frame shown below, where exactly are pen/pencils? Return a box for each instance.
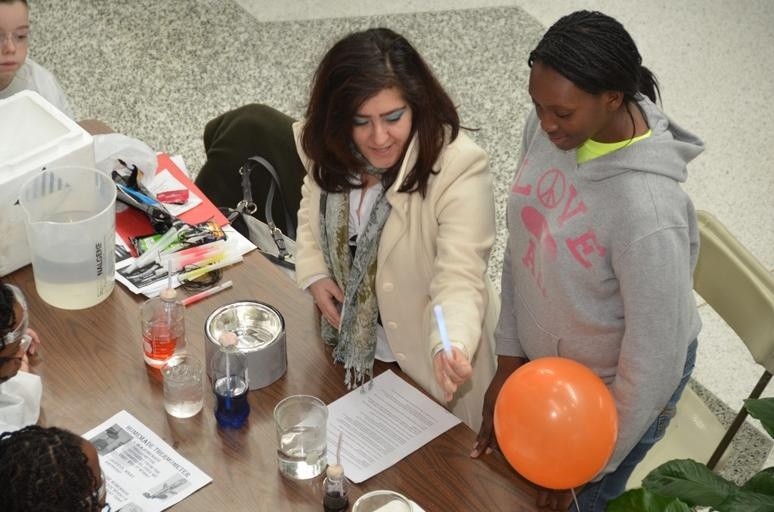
[181,280,232,307]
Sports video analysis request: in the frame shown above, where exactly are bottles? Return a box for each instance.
[209,334,249,429]
[321,464,350,511]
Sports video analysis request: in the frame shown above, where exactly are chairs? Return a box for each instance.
[623,205,774,512]
[190,102,311,263]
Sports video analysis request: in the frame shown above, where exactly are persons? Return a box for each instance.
[0,0,76,123]
[471,10,705,512]
[0,280,43,439]
[0,425,110,512]
[292,29,498,435]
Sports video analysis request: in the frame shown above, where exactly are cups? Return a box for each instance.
[17,166,117,311]
[351,490,414,512]
[138,295,187,368]
[273,393,329,481]
[158,350,203,418]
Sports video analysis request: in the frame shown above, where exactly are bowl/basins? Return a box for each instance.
[201,298,289,391]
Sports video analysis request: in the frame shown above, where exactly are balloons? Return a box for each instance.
[494,357,617,490]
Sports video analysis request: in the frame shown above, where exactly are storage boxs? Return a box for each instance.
[0,85,96,279]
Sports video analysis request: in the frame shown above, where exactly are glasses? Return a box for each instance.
[3,282,30,347]
[80,468,107,508]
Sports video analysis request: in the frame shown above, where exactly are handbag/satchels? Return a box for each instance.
[217,206,296,285]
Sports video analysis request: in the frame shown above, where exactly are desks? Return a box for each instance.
[0,118,579,510]
